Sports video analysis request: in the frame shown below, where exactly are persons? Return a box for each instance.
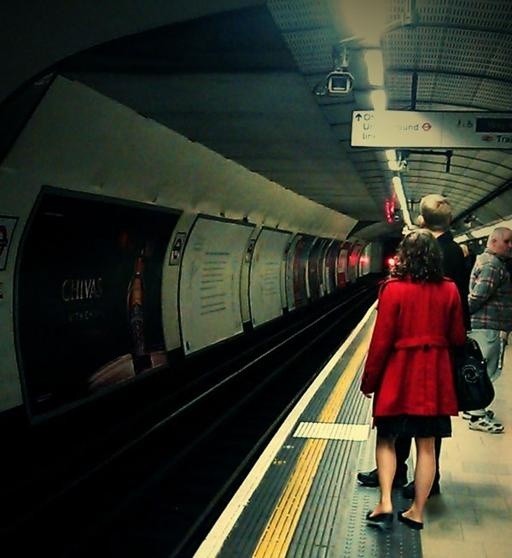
[365,226,468,530]
[355,192,476,502]
[460,225,512,434]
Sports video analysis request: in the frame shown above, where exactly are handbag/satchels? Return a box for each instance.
[450,335,493,409]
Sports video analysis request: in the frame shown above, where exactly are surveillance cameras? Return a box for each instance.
[394,215,400,222]
[327,71,355,94]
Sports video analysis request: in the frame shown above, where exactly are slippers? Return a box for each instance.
[367,511,395,524]
[397,509,424,530]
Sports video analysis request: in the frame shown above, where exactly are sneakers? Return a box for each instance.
[401,478,439,500]
[358,472,406,486]
[462,410,504,433]
